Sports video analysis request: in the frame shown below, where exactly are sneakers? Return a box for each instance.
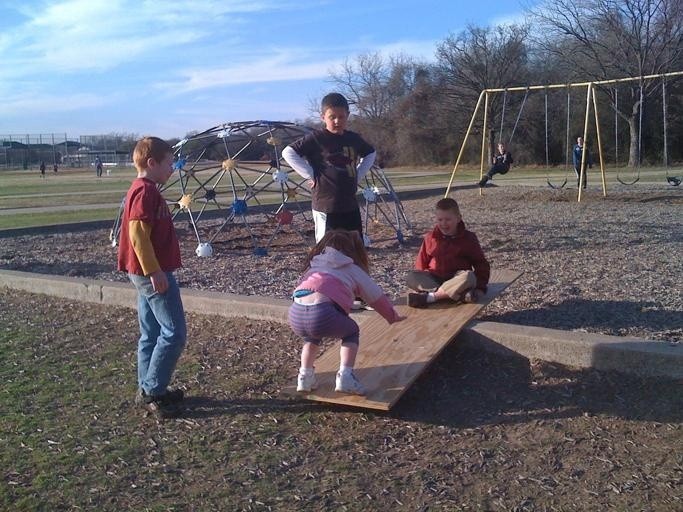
[134,387,181,418]
[166,389,183,403]
[296,366,320,392]
[460,287,478,304]
[406,291,428,308]
[334,371,366,396]
[360,300,374,311]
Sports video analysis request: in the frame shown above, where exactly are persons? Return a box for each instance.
[39,161,46,178]
[53,162,59,173]
[281,92,378,310]
[402,197,491,309]
[288,227,409,396]
[95,154,103,177]
[478,142,514,188]
[115,135,186,419]
[571,136,594,189]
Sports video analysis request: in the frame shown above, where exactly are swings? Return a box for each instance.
[615,80,642,185]
[662,75,683,185]
[544,86,570,189]
[493,90,529,175]
